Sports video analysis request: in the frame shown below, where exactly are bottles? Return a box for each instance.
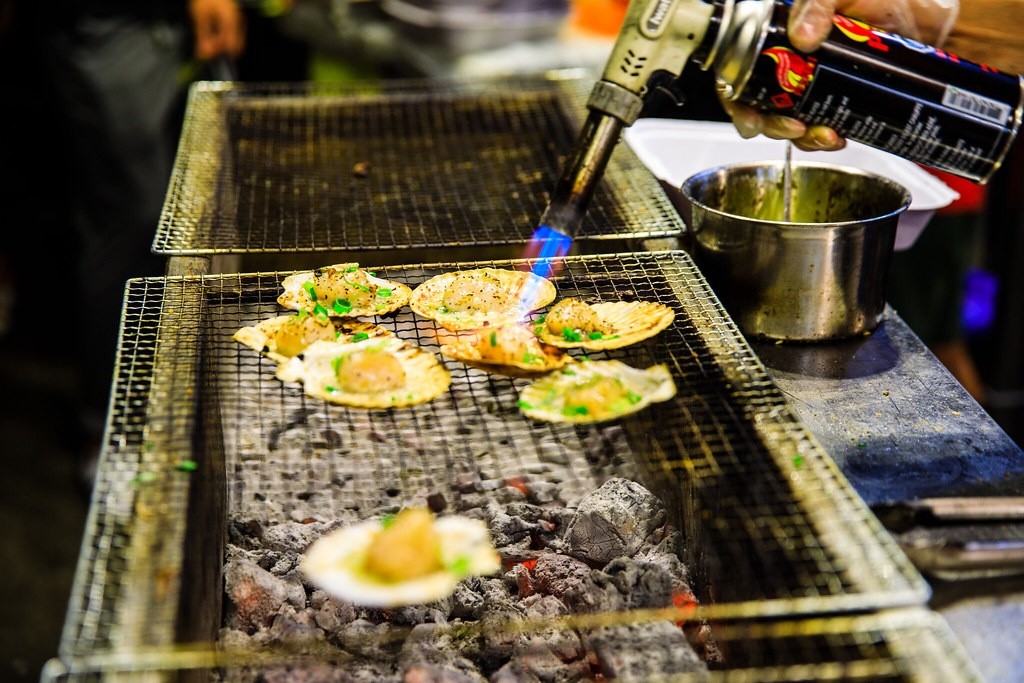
[692,0,1024,186]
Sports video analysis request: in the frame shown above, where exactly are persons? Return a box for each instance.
[720,0,1024,152]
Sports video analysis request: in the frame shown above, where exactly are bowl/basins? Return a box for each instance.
[679,160,913,347]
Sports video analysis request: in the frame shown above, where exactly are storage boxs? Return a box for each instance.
[620,115,962,255]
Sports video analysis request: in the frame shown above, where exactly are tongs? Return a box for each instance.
[872,496,1024,583]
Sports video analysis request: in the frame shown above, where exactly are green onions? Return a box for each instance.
[293,266,644,418]
[381,512,468,580]
